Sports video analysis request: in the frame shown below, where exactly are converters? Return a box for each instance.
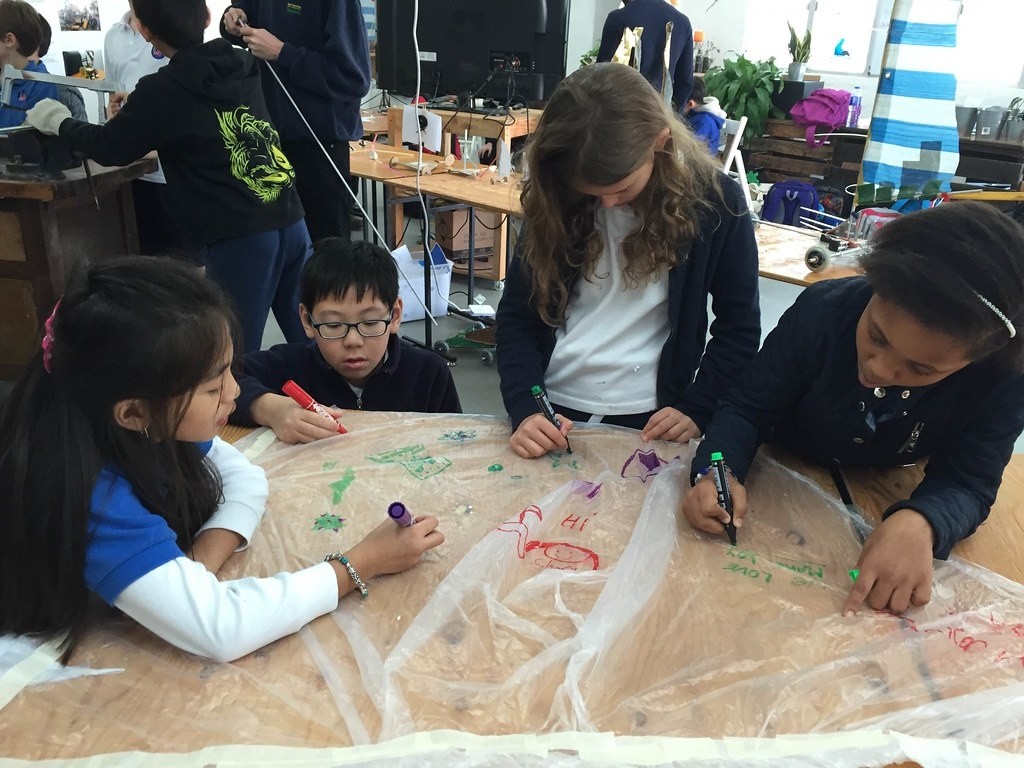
[468,304,496,317]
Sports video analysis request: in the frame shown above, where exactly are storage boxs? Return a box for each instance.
[398,251,454,322]
[432,205,497,259]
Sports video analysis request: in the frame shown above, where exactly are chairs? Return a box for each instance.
[720,116,755,212]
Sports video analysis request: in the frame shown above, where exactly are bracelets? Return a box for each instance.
[324,550,368,598]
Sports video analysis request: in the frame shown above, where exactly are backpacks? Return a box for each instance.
[763,180,819,227]
[788,86,861,146]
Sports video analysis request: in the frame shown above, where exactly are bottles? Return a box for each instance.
[845,87,862,128]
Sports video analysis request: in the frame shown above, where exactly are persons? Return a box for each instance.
[228,237,463,448]
[596,0,694,112]
[494,61,762,459]
[684,76,727,156]
[219,0,371,248]
[104,0,171,119]
[681,200,1024,619]
[0,0,58,129]
[0,254,446,662]
[28,0,314,353]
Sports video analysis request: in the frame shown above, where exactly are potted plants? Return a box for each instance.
[785,22,815,82]
[712,59,805,128]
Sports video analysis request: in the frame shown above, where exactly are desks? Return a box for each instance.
[343,140,868,368]
[0,405,1023,767]
[0,143,168,401]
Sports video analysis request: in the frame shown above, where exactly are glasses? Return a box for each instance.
[305,308,395,340]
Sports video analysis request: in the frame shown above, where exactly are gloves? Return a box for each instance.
[27,98,72,135]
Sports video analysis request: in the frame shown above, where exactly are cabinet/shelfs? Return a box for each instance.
[382,105,545,292]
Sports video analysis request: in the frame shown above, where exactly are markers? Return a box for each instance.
[281,379,348,434]
[386,501,417,531]
[531,385,573,455]
[711,452,739,547]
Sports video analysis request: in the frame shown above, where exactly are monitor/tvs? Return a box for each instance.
[376,0,571,115]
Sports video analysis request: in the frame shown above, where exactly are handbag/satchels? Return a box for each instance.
[402,105,442,157]
